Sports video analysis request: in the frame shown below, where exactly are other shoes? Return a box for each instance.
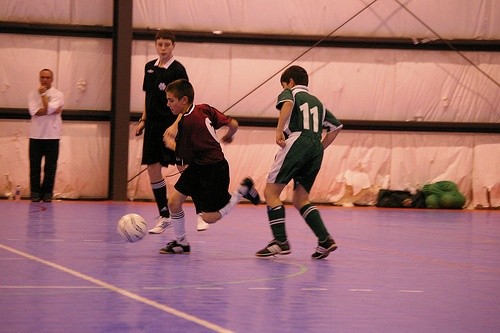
[31,192,41,202]
[43,193,53,202]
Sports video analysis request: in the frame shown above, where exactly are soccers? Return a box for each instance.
[117,214,148,242]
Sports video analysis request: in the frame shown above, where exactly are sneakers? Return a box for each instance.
[256,239,291,255]
[160,240,190,254]
[312,235,337,259]
[148,215,174,233]
[238,176,259,205]
[197,213,209,231]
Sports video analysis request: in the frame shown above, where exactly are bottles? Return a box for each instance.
[15,185,21,200]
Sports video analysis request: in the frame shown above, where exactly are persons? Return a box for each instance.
[135,31,210,234]
[256,65,343,260]
[28,68,63,202]
[158,79,260,254]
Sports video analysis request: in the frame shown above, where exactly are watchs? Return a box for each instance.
[139,118,145,122]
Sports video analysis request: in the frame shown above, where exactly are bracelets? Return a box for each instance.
[41,93,46,96]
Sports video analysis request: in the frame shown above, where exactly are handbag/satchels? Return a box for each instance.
[376,189,425,208]
[422,181,465,209]
